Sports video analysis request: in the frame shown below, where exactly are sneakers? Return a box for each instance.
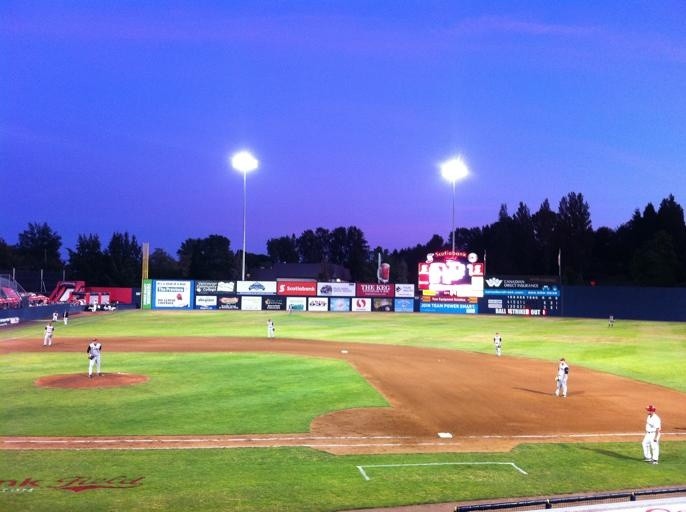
[643,458,658,465]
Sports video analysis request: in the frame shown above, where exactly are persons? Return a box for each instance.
[50,309,58,325]
[642,404,662,464]
[86,337,102,378]
[266,318,276,339]
[62,308,69,326]
[493,331,503,357]
[555,357,570,398]
[42,320,55,347]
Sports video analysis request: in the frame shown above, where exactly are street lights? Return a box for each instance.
[440,158,465,252]
[230,150,257,282]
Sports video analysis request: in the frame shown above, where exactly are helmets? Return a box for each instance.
[646,404,656,412]
